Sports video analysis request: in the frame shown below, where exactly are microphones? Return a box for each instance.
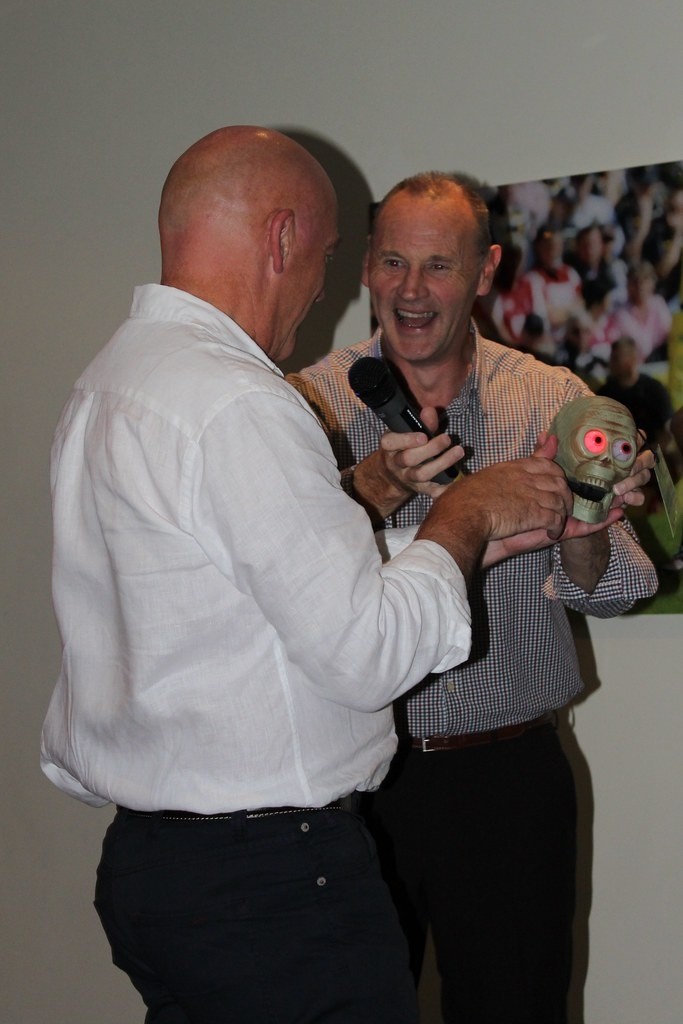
[348,357,463,485]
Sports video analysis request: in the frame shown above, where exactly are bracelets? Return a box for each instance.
[340,464,356,500]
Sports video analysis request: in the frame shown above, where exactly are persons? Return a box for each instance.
[267,172,656,1024]
[494,169,683,615]
[40,126,622,1022]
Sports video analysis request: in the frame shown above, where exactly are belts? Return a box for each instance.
[110,793,351,819]
[395,712,559,752]
